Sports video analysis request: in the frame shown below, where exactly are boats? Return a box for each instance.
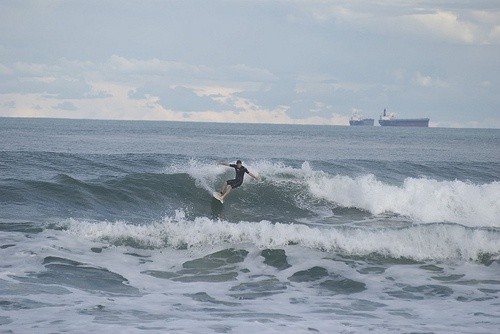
[348,109,374,126]
[378,107,430,127]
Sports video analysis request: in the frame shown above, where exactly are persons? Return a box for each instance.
[219,160,257,200]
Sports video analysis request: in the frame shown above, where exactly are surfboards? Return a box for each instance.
[213,191,224,205]
